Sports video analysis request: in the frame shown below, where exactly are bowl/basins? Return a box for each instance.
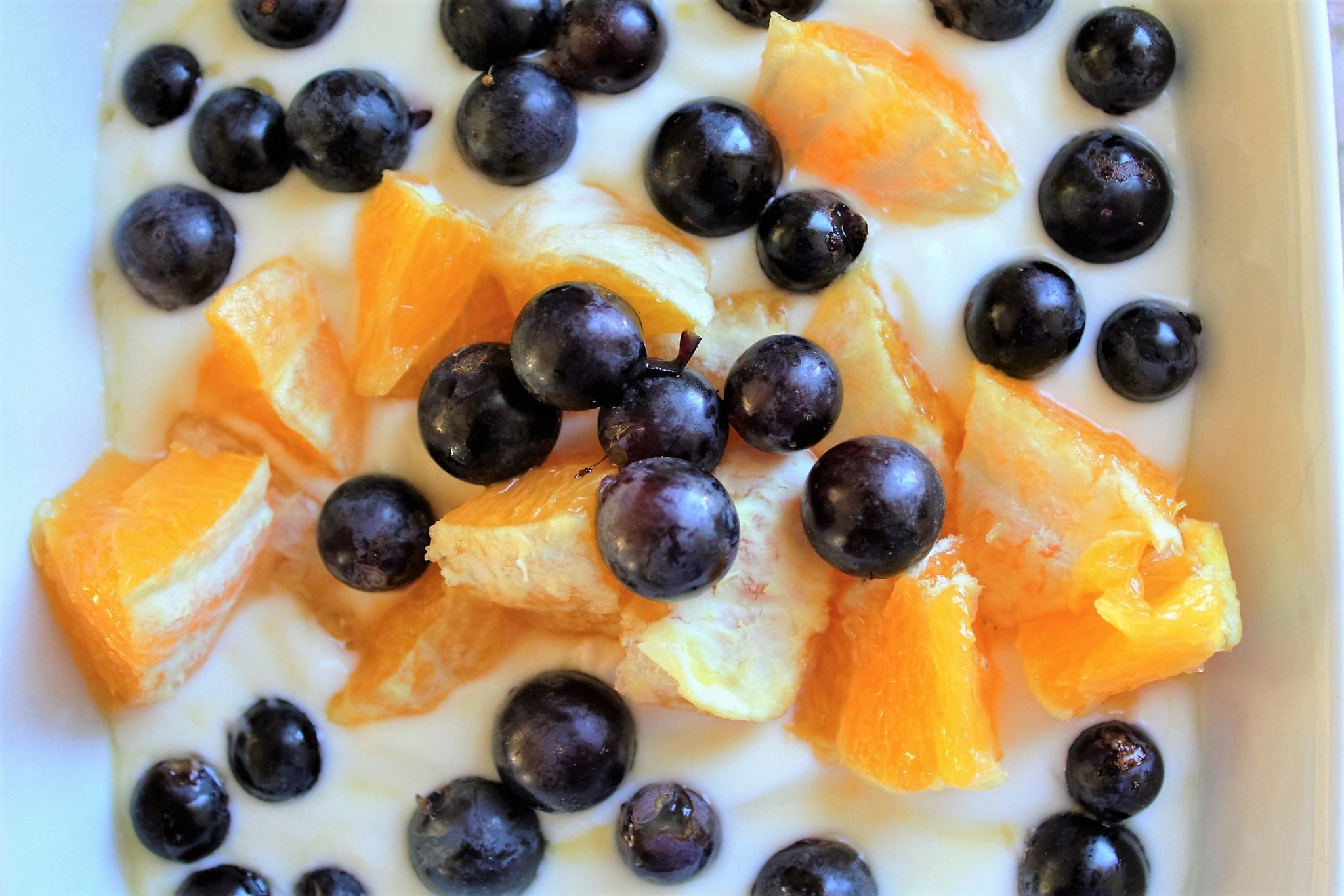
[2,0,1344,896]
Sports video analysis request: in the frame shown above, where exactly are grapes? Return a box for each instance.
[120,0,1208,896]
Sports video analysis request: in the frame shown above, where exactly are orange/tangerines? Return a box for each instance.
[27,21,1246,789]
